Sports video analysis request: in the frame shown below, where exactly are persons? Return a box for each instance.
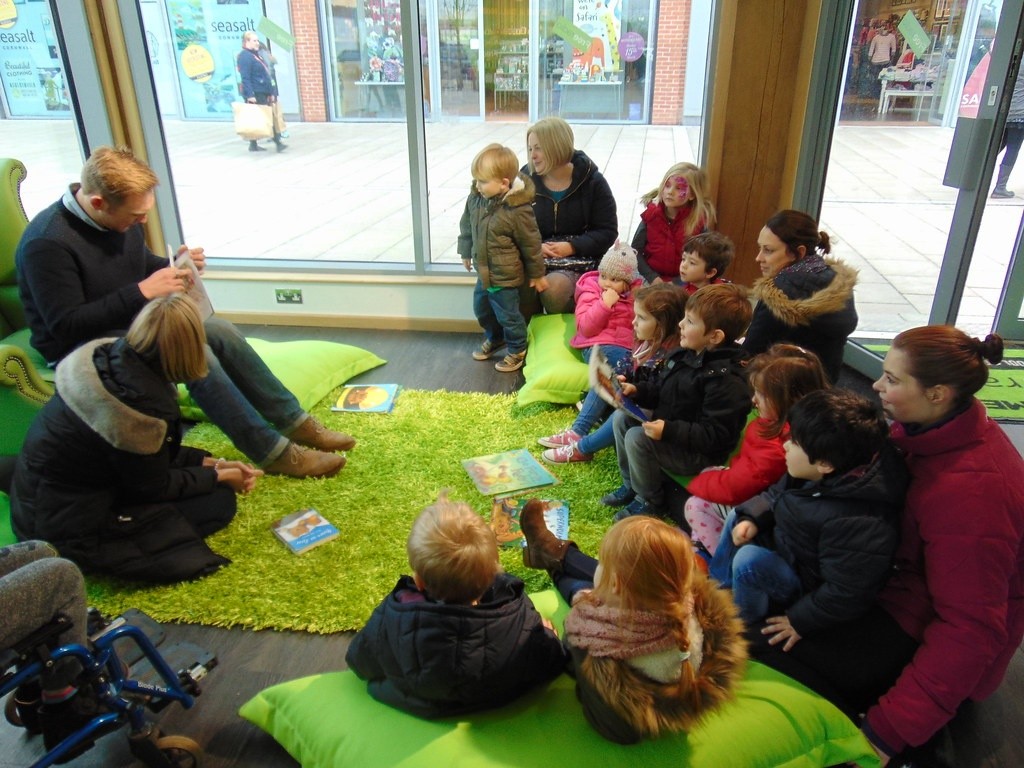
[0,539,129,763]
[346,121,1024,768]
[15,147,355,480]
[256,41,290,138]
[869,31,896,70]
[990,51,1024,197]
[238,30,288,153]
[9,292,263,585]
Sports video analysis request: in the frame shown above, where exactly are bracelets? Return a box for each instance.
[214,457,225,470]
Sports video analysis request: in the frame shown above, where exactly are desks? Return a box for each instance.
[355,81,405,117]
[558,81,623,120]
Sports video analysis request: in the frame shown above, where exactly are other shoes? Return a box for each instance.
[281,131,289,138]
[277,142,288,152]
[13,686,137,764]
[249,146,266,151]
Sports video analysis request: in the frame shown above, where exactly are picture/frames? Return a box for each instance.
[930,0,968,44]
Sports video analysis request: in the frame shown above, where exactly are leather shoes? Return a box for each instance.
[602,485,654,521]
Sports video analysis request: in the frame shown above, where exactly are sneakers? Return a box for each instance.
[538,430,593,465]
[286,415,355,451]
[264,442,346,479]
[495,349,527,371]
[472,338,507,360]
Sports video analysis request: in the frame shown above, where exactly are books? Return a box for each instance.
[331,383,398,414]
[493,459,561,501]
[490,496,570,546]
[270,509,339,554]
[462,448,554,495]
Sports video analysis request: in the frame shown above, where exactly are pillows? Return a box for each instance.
[177,337,388,419]
[239,590,882,768]
[517,311,589,408]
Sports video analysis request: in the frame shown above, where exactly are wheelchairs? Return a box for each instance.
[0,605,219,768]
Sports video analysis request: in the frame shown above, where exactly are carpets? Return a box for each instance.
[866,339,1024,424]
[74,384,673,633]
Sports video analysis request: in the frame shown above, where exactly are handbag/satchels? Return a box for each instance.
[231,102,274,138]
[268,100,286,133]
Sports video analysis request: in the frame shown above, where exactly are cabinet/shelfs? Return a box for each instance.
[879,78,940,120]
[493,51,528,110]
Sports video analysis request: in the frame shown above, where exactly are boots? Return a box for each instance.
[519,498,579,578]
[991,164,1014,198]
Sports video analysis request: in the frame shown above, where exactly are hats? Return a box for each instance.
[598,239,639,285]
